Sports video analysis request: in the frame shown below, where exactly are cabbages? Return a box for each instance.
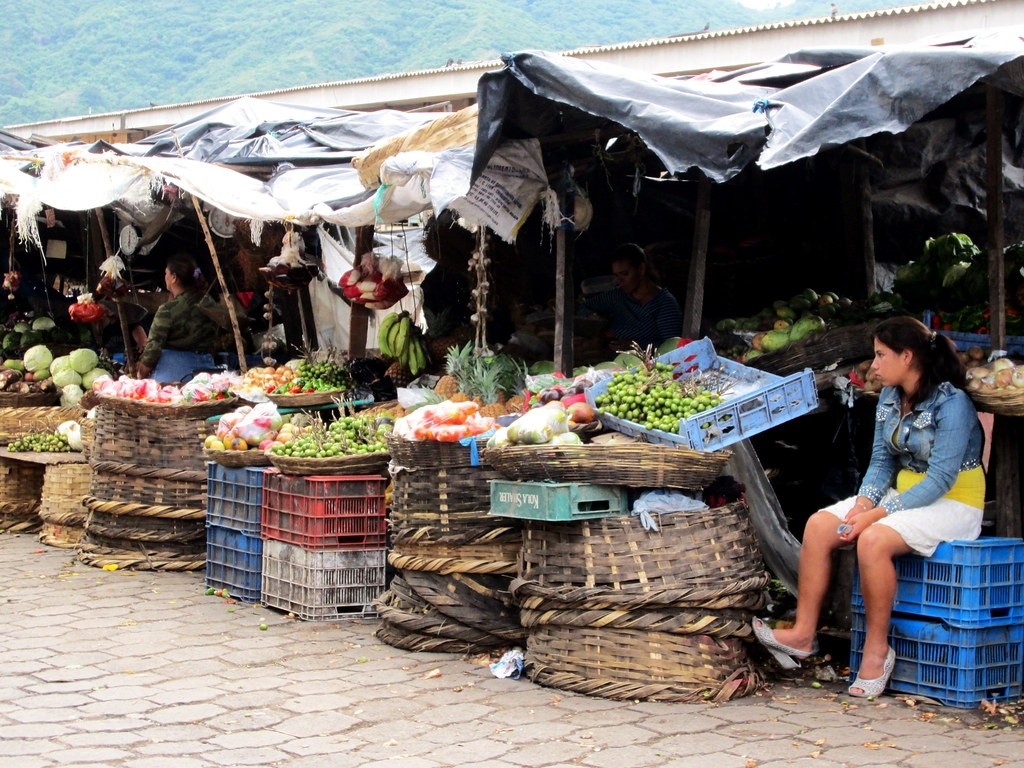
[55,420,82,452]
[892,231,1024,301]
[0,317,113,406]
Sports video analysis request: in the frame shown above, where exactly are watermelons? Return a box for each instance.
[530,337,682,373]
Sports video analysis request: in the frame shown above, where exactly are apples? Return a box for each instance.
[206,412,313,449]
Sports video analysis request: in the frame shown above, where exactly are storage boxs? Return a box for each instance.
[585,335,820,449]
[852,537,1024,629]
[205,525,263,604]
[851,617,1024,708]
[205,460,266,538]
[922,309,1024,356]
[487,477,629,521]
[261,466,387,551]
[260,538,386,622]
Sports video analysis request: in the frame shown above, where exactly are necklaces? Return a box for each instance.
[903,396,907,415]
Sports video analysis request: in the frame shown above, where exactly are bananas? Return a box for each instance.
[378,310,426,375]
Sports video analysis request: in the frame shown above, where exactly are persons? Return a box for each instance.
[136,257,223,385]
[751,316,985,698]
[97,294,147,351]
[583,242,682,356]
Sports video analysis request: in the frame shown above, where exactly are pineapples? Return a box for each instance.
[383,337,457,386]
[435,341,525,418]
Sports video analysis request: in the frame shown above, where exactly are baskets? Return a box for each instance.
[0,323,1024,704]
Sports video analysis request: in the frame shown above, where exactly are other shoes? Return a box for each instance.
[752,616,814,669]
[848,645,896,697]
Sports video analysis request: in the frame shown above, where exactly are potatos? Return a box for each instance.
[856,360,881,392]
[958,346,1024,392]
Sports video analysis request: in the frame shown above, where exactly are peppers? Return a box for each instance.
[933,304,1024,337]
[93,371,231,402]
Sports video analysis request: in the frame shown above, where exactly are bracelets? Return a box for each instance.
[855,502,867,511]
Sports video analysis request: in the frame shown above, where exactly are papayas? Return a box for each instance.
[717,289,907,362]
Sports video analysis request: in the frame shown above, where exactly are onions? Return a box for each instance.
[244,365,298,388]
